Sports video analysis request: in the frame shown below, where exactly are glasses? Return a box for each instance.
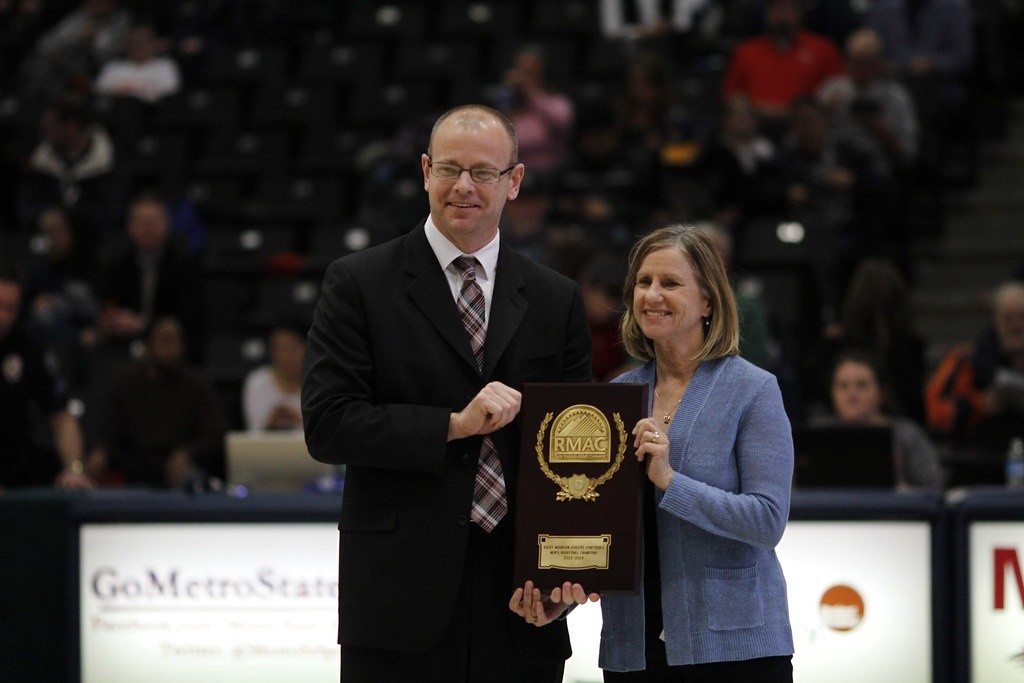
[424,156,514,186]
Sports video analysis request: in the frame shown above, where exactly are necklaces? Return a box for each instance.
[653,388,681,424]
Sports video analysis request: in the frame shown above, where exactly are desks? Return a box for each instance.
[0,480,1024,683]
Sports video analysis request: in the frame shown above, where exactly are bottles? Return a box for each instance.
[1007,440,1024,486]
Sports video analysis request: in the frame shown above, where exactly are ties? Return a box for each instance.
[456,255,507,533]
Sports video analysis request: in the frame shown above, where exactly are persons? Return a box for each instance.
[300,103,595,683]
[0,0,1024,498]
[549,225,794,683]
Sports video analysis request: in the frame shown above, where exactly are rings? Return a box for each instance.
[652,432,659,442]
[531,616,538,622]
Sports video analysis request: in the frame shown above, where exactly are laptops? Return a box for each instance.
[789,425,898,491]
[226,432,334,497]
